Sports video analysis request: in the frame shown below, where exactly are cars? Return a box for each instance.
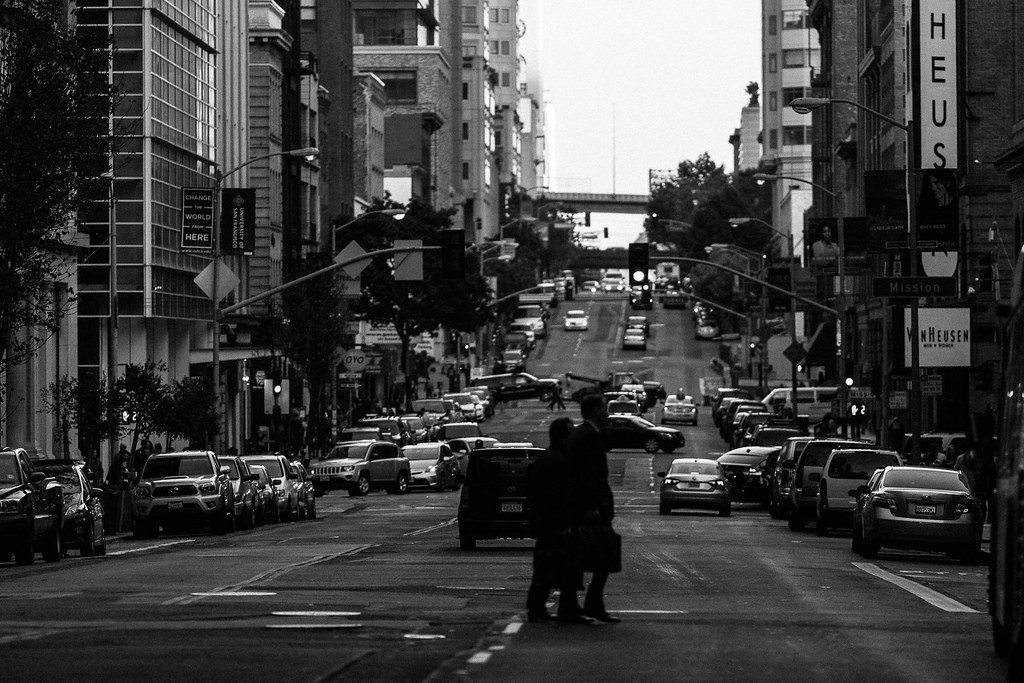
[605,370,698,454]
[497,262,721,370]
[847,466,983,564]
[130,448,316,537]
[656,457,732,516]
[0,446,105,565]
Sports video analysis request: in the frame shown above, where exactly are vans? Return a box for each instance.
[762,387,850,422]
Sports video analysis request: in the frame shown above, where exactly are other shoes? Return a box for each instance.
[528,610,557,622]
[581,608,621,624]
[559,612,594,624]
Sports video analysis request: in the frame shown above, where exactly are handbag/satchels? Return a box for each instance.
[583,527,622,573]
[534,530,585,591]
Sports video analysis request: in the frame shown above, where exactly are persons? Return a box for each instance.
[565,280,575,301]
[82,438,239,490]
[283,353,1024,522]
[526,393,623,626]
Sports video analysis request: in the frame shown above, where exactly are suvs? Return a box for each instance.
[307,367,558,498]
[715,388,903,530]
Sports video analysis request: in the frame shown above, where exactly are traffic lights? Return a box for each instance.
[844,361,854,386]
[272,367,283,398]
[122,409,136,425]
[749,342,755,357]
[628,243,648,285]
[851,401,866,416]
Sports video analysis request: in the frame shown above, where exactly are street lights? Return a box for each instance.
[728,216,798,411]
[753,172,847,376]
[329,209,401,443]
[215,145,320,451]
[788,92,922,460]
